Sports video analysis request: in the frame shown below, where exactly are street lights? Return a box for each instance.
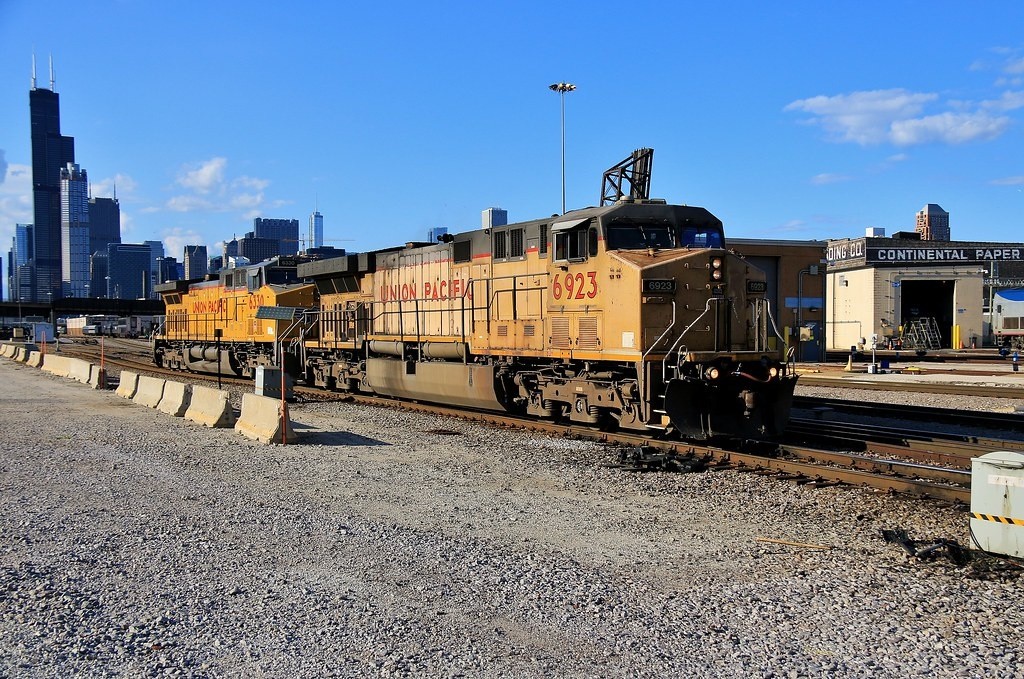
[548,82,578,218]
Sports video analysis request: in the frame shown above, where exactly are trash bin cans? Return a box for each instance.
[969,336,977,348]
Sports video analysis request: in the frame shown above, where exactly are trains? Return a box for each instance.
[152,192,801,445]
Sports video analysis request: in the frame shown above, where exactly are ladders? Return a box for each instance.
[908,317,943,350]
